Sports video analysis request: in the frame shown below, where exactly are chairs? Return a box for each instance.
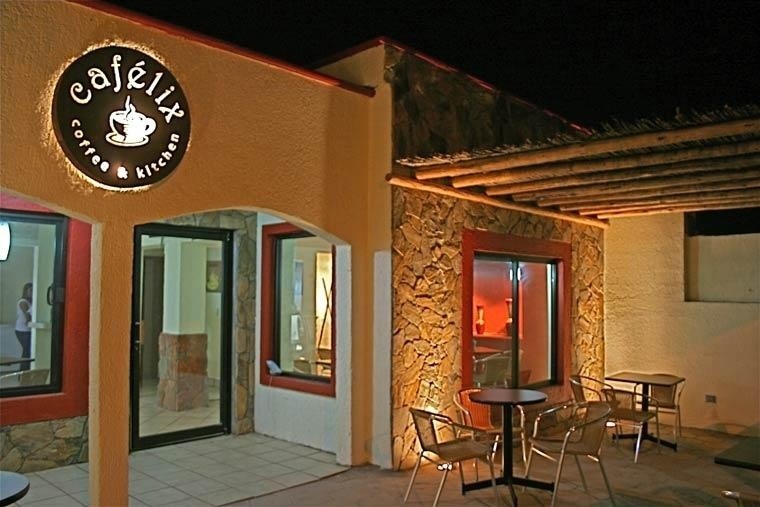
[632,373,682,446]
[600,388,662,464]
[403,407,503,506]
[567,373,624,448]
[453,387,529,482]
[522,400,616,506]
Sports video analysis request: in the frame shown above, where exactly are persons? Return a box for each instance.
[15,283,33,380]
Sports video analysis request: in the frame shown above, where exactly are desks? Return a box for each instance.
[605,371,685,455]
[714,435,760,471]
[461,388,556,506]
